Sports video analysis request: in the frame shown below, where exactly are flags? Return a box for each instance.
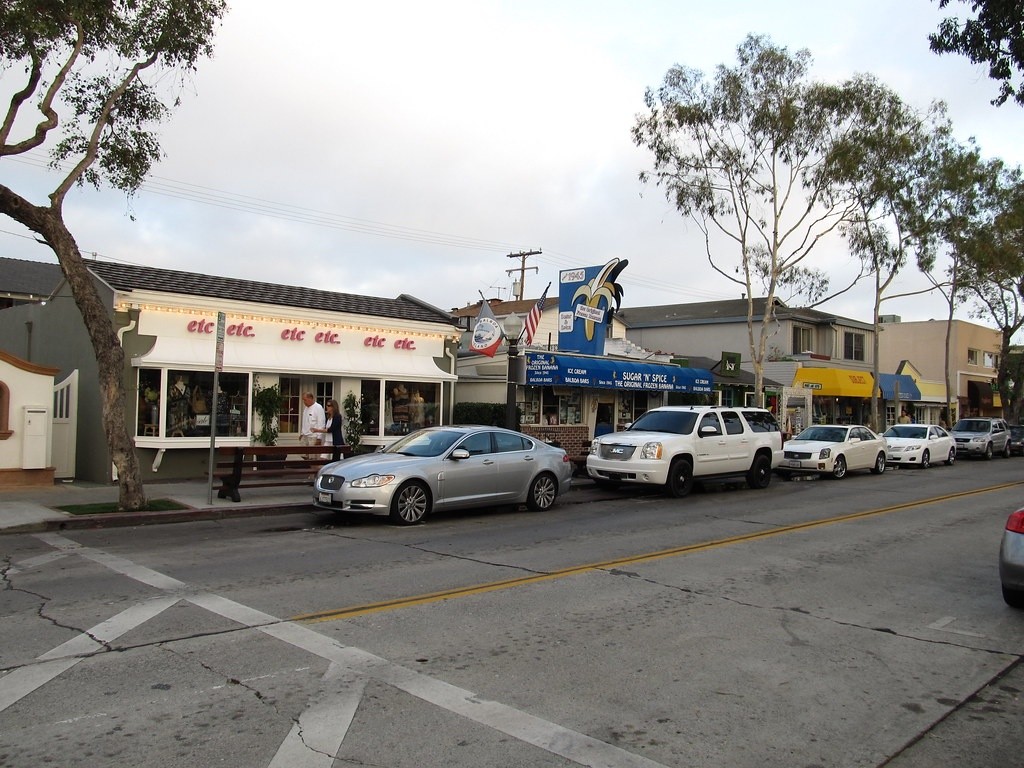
[524,286,546,347]
[469,299,503,359]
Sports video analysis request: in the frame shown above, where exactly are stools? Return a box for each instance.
[143,423,159,436]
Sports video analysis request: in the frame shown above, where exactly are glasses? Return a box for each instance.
[327,405,332,406]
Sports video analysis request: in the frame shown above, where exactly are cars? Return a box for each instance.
[312,425,572,526]
[776,422,889,477]
[1006,424,1024,456]
[878,424,957,469]
[949,418,1012,459]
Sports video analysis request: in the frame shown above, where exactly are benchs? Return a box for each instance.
[206,444,351,503]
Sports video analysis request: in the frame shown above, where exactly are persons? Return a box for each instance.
[898,410,911,424]
[299,392,326,480]
[310,399,345,470]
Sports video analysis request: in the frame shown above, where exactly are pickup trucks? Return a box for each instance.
[586,403,784,500]
